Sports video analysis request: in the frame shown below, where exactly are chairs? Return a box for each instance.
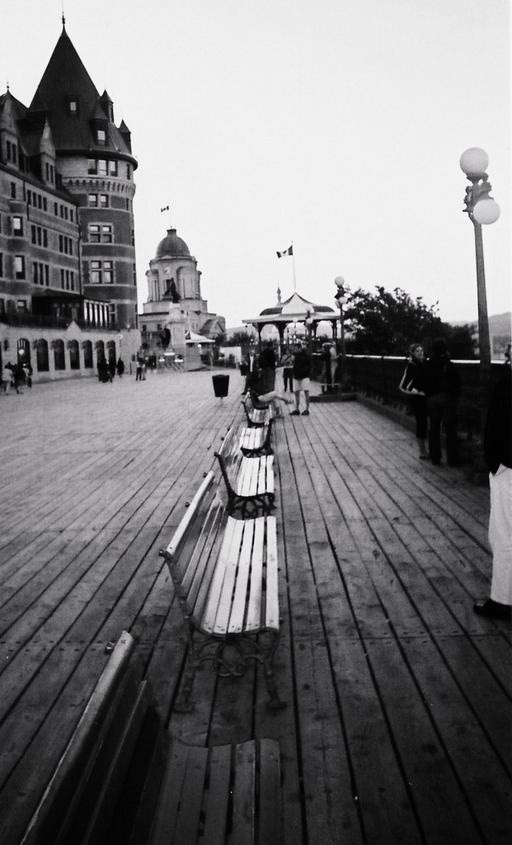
[154,469,283,650]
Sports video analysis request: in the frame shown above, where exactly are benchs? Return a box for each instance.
[212,392,282,514]
[18,623,283,843]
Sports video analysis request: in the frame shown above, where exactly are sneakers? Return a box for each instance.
[289,409,310,416]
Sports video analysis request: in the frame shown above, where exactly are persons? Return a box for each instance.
[237,339,343,417]
[473,344,511,624]
[13,363,27,395]
[99,356,155,383]
[397,343,443,465]
[2,364,13,394]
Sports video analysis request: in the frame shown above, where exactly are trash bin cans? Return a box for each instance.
[212,374,229,400]
[238,364,248,378]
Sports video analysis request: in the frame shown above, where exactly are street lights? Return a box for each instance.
[304,305,314,367]
[334,275,346,356]
[460,146,500,485]
